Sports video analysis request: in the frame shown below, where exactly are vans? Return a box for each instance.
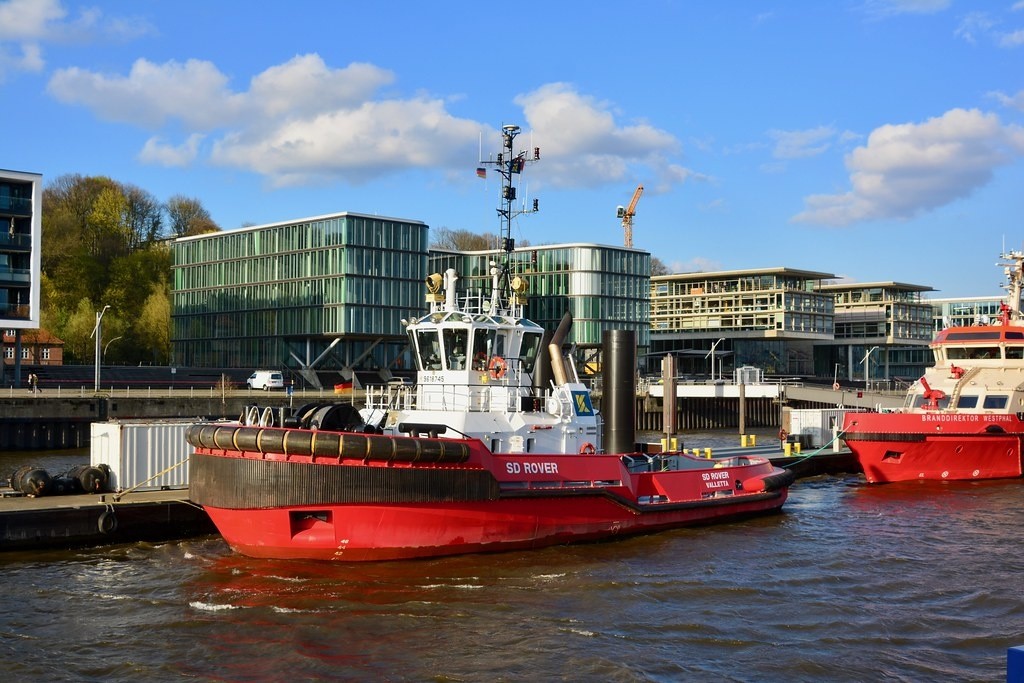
[246,370,285,392]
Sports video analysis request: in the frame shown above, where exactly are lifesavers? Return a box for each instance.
[580,443,595,455]
[833,383,841,390]
[779,429,787,441]
[474,353,488,370]
[490,356,506,378]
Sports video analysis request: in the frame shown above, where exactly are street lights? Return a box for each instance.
[704,337,726,380]
[764,377,802,450]
[102,335,123,366]
[860,346,880,392]
[647,376,688,453]
[89,305,112,392]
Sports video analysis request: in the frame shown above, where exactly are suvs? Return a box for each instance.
[383,376,414,390]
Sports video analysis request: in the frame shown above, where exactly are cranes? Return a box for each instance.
[616,183,645,247]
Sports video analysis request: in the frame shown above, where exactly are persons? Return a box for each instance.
[27,374,42,393]
[428,353,437,366]
[396,356,402,369]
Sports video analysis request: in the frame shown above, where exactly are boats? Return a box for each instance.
[835,235,1024,486]
[182,121,796,562]
